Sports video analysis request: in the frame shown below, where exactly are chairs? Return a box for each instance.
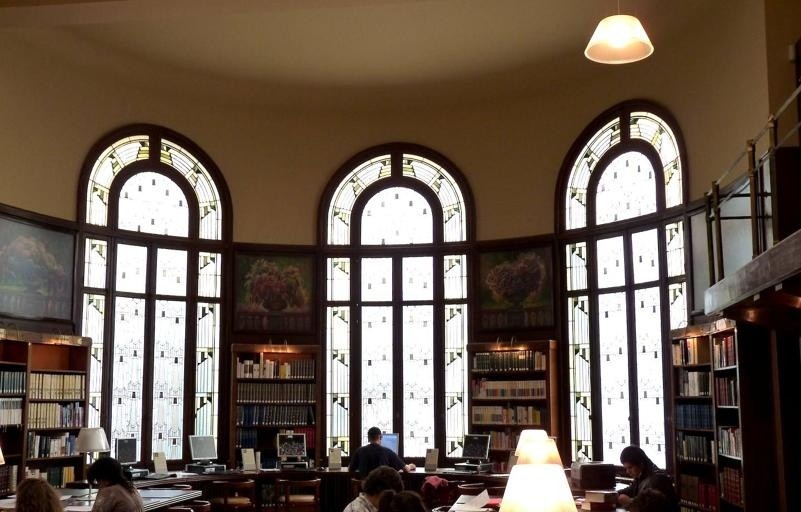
[458,483,506,496]
[167,499,212,512]
[350,478,362,499]
[274,477,321,512]
[147,484,192,490]
[432,505,451,512]
[207,478,255,512]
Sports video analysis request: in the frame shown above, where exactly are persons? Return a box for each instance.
[87,457,143,512]
[342,465,404,512]
[377,490,427,512]
[626,487,670,512]
[0,478,62,511]
[347,426,416,480]
[615,444,682,512]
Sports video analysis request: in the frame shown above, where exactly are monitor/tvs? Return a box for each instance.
[277,433,307,461]
[462,434,490,464]
[381,433,399,456]
[115,438,137,469]
[188,434,218,465]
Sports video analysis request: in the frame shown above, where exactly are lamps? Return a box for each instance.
[497,428,579,512]
[74,427,111,464]
[584,0,654,65]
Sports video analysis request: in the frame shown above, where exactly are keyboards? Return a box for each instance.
[442,470,477,474]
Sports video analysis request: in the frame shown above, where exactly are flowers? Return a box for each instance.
[243,259,303,307]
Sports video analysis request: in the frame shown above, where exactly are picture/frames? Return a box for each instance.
[0,200,79,336]
[232,248,316,337]
[474,233,557,342]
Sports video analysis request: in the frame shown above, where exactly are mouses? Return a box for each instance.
[319,466,324,470]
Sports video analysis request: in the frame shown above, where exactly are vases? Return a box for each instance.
[262,296,288,312]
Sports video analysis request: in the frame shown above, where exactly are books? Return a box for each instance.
[471,350,547,473]
[236,355,316,507]
[671,334,741,512]
[580,490,618,511]
[0,365,86,498]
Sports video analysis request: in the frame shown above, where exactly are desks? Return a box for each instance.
[66,464,634,493]
[0,490,203,512]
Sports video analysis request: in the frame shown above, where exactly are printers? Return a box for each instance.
[571,461,616,489]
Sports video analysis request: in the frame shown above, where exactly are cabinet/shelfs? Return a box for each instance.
[670,319,768,511]
[467,340,558,473]
[0,329,92,497]
[231,344,322,469]
[685,145,801,315]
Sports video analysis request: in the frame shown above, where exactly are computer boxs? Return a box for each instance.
[185,464,226,473]
[129,469,149,481]
[454,463,494,472]
[276,461,308,471]
